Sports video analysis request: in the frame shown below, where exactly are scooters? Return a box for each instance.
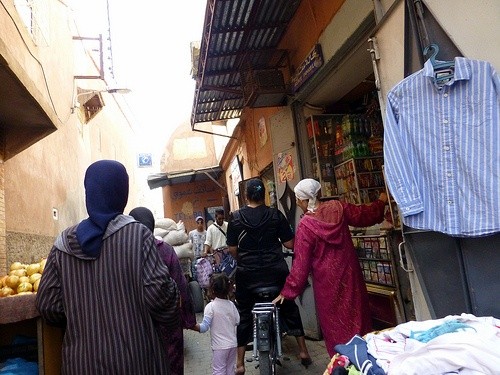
[246,249,302,375]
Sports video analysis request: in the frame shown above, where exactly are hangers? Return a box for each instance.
[423,44,455,83]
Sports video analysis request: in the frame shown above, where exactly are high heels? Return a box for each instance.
[235,366,246,375]
[299,352,311,369]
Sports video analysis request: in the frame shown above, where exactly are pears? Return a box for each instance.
[0,259,47,297]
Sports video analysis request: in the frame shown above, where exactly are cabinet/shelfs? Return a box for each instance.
[307,113,410,325]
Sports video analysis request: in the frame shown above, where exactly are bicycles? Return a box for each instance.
[206,247,236,276]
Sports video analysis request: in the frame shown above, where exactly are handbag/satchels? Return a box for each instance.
[195,257,213,290]
[216,252,238,279]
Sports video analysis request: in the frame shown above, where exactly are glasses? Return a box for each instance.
[217,217,224,220]
[196,221,203,224]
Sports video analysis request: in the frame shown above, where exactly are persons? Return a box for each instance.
[189,210,236,301]
[271,179,388,364]
[192,271,241,375]
[128,208,196,375]
[35,160,183,375]
[227,180,313,375]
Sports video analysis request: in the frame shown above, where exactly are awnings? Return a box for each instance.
[189,0,302,138]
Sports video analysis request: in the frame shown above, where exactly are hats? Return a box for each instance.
[195,216,204,221]
[216,208,224,213]
